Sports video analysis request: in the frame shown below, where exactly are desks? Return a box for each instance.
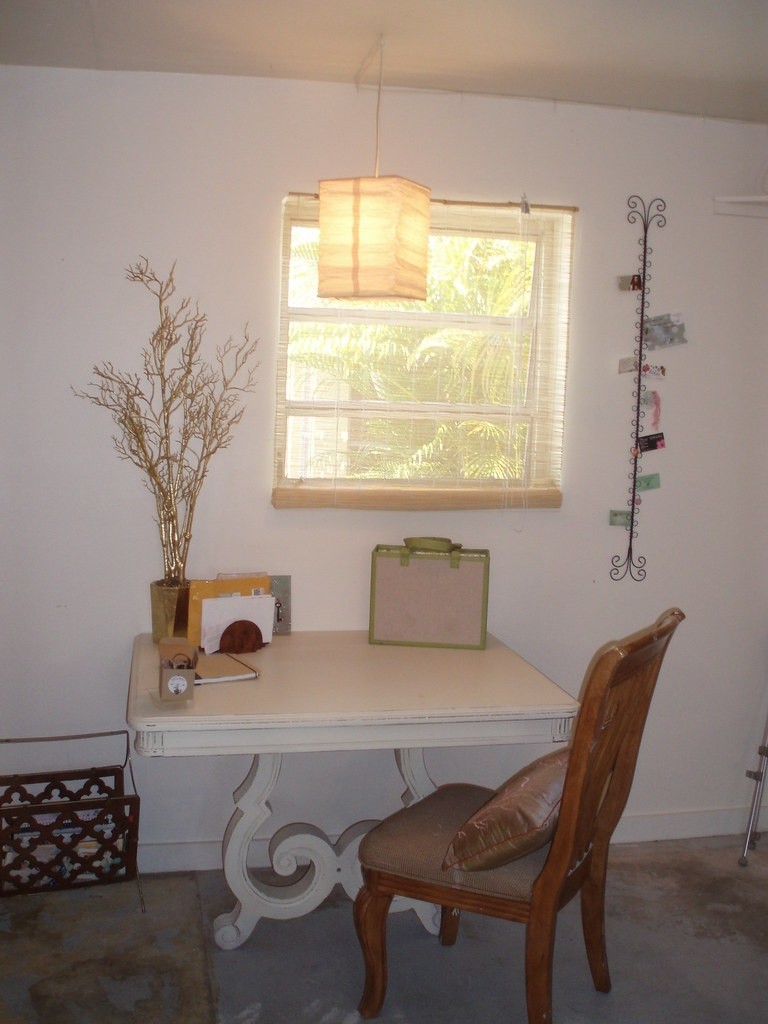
[127,632,580,952]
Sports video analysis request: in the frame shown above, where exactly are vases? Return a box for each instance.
[150,578,191,643]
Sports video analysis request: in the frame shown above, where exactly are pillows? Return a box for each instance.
[443,745,571,872]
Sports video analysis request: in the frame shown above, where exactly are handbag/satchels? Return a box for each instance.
[368,535,489,650]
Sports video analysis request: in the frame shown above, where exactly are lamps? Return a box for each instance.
[317,39,430,300]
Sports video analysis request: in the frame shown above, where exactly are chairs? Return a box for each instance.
[351,608,687,1024]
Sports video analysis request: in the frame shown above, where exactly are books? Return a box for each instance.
[194,652,262,684]
